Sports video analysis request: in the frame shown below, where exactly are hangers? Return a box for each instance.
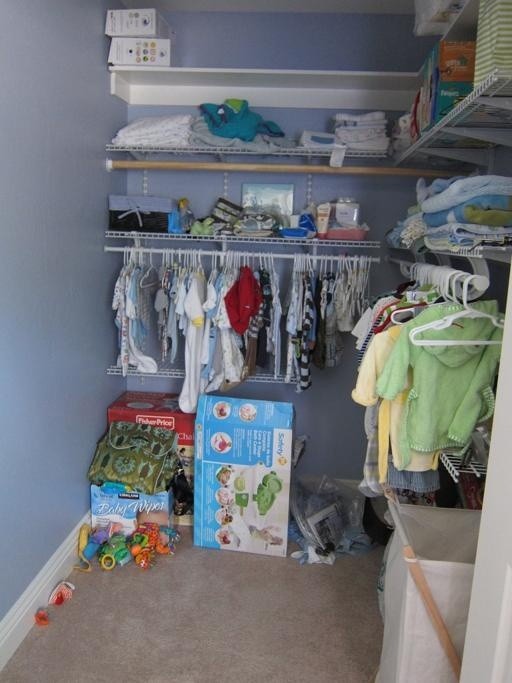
[391,261,505,346]
[211,250,275,274]
[118,246,159,288]
[161,248,204,278]
[293,252,372,282]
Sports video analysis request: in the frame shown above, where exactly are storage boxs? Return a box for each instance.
[108,38,177,65]
[105,7,177,39]
[193,395,298,558]
[105,392,195,530]
[90,482,173,536]
[472,0,511,89]
[108,195,180,233]
[417,40,475,137]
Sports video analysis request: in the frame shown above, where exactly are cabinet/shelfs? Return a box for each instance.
[390,2,512,483]
[106,61,420,387]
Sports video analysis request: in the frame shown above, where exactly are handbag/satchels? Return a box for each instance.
[88,420,178,496]
